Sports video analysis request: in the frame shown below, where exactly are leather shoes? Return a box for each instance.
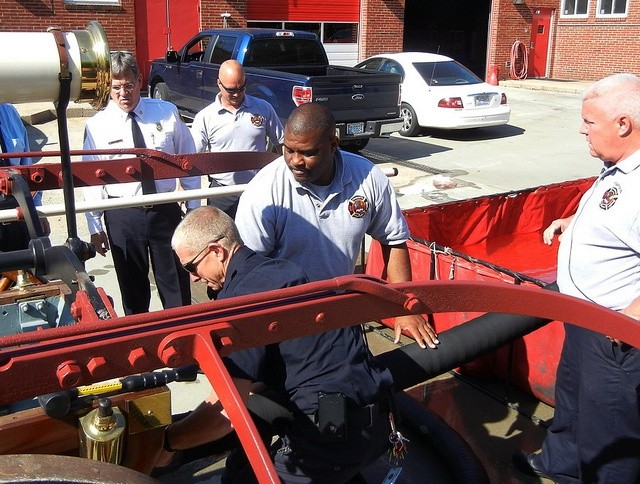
[512,448,555,481]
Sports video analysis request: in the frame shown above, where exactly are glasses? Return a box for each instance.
[182,235,225,273]
[218,77,248,94]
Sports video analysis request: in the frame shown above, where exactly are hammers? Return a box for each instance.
[37,364,197,418]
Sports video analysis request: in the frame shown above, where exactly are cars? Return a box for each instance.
[351,52,510,137]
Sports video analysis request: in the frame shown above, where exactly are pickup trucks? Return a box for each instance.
[364,173,570,427]
[148,29,405,153]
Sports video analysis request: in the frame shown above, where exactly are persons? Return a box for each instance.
[82,51,201,317]
[171,203,396,484]
[233,102,440,348]
[0,104,42,205]
[190,59,285,301]
[512,73,640,482]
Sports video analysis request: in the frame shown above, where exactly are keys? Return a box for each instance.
[388,413,409,468]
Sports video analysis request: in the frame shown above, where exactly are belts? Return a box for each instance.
[107,195,174,209]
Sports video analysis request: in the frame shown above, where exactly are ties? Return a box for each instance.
[127,111,158,195]
